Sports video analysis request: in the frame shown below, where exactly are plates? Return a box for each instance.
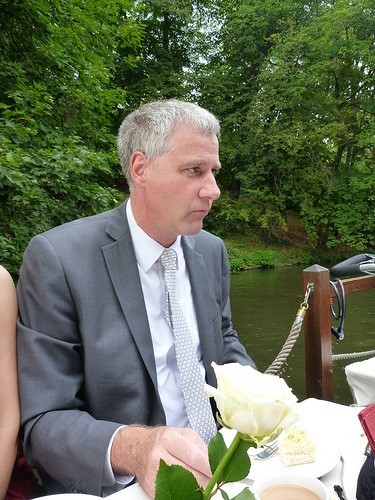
[31,493,109,500]
[218,403,342,480]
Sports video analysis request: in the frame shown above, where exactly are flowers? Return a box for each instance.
[153,358,304,500]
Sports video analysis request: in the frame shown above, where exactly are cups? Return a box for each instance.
[251,471,329,500]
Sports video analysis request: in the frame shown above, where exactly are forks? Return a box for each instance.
[250,440,279,460]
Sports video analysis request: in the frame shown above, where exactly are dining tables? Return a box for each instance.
[103,396,375,500]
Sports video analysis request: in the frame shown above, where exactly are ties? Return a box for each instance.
[163,249,218,447]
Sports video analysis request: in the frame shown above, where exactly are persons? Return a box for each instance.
[17,97,265,500]
[0,265,43,500]
